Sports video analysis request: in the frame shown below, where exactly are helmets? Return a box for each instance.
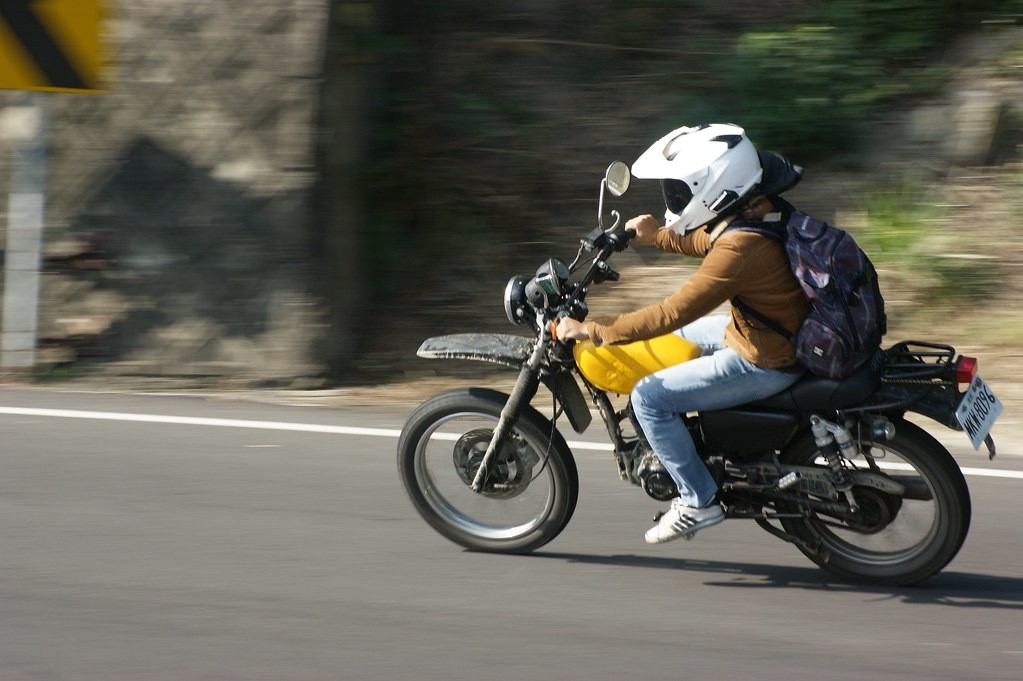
[630,122,763,234]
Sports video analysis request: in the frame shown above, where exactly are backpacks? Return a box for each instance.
[711,197,886,379]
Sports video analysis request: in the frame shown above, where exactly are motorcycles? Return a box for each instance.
[396,162,1005,586]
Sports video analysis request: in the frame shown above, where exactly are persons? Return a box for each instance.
[556,122,810,544]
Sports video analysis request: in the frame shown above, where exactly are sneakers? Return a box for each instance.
[646,498,723,544]
